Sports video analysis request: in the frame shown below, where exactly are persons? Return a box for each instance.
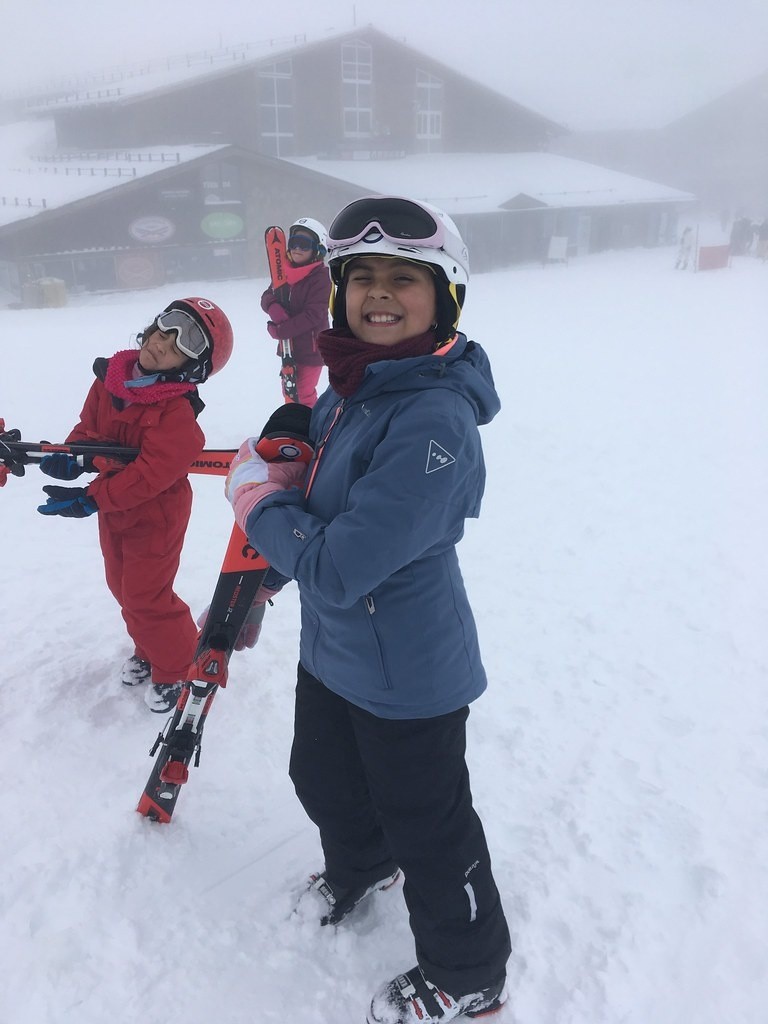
[34,296,235,714]
[675,208,768,270]
[197,196,513,1024]
[261,216,335,406]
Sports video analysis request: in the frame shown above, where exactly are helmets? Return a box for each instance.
[288,218,328,254]
[163,297,234,383]
[326,195,470,342]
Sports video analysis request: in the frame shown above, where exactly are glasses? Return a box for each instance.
[155,309,211,364]
[327,195,469,279]
[288,235,326,254]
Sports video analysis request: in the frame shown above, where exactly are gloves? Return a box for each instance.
[39,440,86,481]
[225,437,306,538]
[268,302,289,324]
[267,321,279,339]
[38,485,99,519]
[197,584,282,651]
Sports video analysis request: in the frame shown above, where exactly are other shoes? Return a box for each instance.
[145,683,185,713]
[121,655,151,686]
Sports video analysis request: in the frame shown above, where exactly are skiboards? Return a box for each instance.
[263,222,299,398]
[135,401,321,823]
[0,420,239,484]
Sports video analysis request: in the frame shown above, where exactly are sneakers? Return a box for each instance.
[295,866,401,926]
[367,965,508,1024]
